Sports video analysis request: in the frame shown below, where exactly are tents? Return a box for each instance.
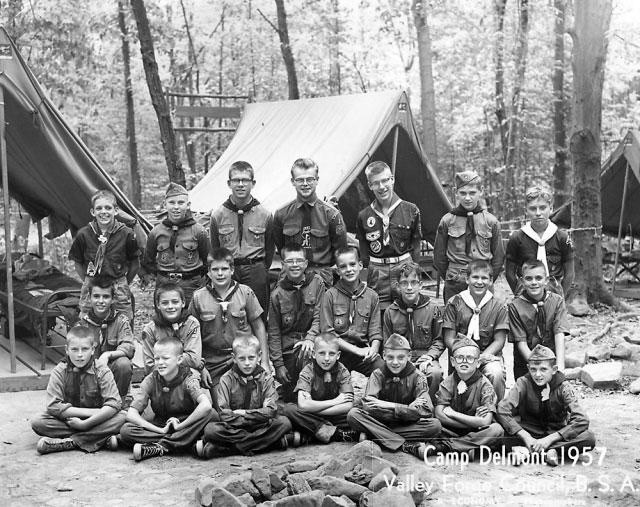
[0,25,155,372]
[550,129,640,298]
[161,91,458,262]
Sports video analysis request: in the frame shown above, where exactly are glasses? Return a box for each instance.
[286,259,304,265]
[368,177,394,186]
[294,178,316,185]
[400,279,420,288]
[454,356,481,365]
[230,179,255,187]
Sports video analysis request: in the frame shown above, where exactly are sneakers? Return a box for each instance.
[133,443,168,463]
[512,446,529,467]
[465,449,477,460]
[401,442,430,460]
[105,436,118,453]
[276,434,290,450]
[201,442,231,456]
[430,442,448,454]
[292,430,310,448]
[191,440,202,457]
[38,438,75,454]
[339,428,362,444]
[546,448,559,467]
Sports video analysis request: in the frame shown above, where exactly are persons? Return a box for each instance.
[285,332,367,443]
[382,261,445,406]
[31,326,127,453]
[141,282,201,374]
[505,186,573,299]
[274,158,346,287]
[209,160,273,329]
[267,243,325,401]
[442,260,508,408]
[494,344,596,465]
[141,182,209,313]
[203,335,300,457]
[68,190,139,331]
[320,247,383,377]
[355,161,421,346]
[188,247,275,411]
[508,260,565,382]
[120,338,213,461]
[74,275,135,407]
[434,172,504,304]
[426,333,504,461]
[347,333,441,458]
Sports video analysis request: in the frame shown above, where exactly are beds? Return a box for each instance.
[0,252,136,371]
[603,249,640,282]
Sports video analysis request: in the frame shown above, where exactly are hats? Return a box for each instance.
[530,344,558,361]
[452,172,482,186]
[384,335,412,349]
[165,183,185,198]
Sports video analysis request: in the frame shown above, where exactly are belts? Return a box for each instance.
[367,255,412,263]
